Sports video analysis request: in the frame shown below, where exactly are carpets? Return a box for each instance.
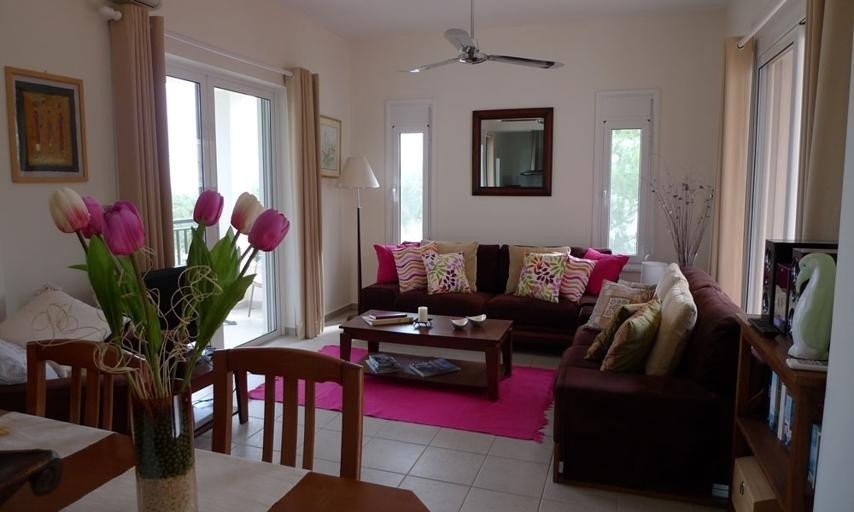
[247,344,558,443]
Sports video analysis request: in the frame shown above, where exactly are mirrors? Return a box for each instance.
[471,107,553,197]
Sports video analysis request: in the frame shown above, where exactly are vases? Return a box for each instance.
[128,377,200,511]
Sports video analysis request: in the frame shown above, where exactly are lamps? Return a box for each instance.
[333,156,380,322]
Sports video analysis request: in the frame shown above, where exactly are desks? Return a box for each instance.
[0,409,430,511]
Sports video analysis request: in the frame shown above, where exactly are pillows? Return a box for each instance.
[0,281,132,386]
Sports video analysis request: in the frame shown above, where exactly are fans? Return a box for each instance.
[391,1,566,77]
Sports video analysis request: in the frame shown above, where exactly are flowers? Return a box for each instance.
[46,187,290,424]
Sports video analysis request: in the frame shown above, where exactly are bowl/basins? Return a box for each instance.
[468,314,487,326]
[451,318,469,329]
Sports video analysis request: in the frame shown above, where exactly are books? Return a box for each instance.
[367,311,408,320]
[364,352,402,376]
[361,316,415,326]
[766,370,822,492]
[407,356,462,378]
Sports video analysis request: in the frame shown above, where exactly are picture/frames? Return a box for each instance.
[319,115,341,179]
[3,65,89,184]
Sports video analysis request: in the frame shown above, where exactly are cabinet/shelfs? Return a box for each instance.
[729,313,827,512]
